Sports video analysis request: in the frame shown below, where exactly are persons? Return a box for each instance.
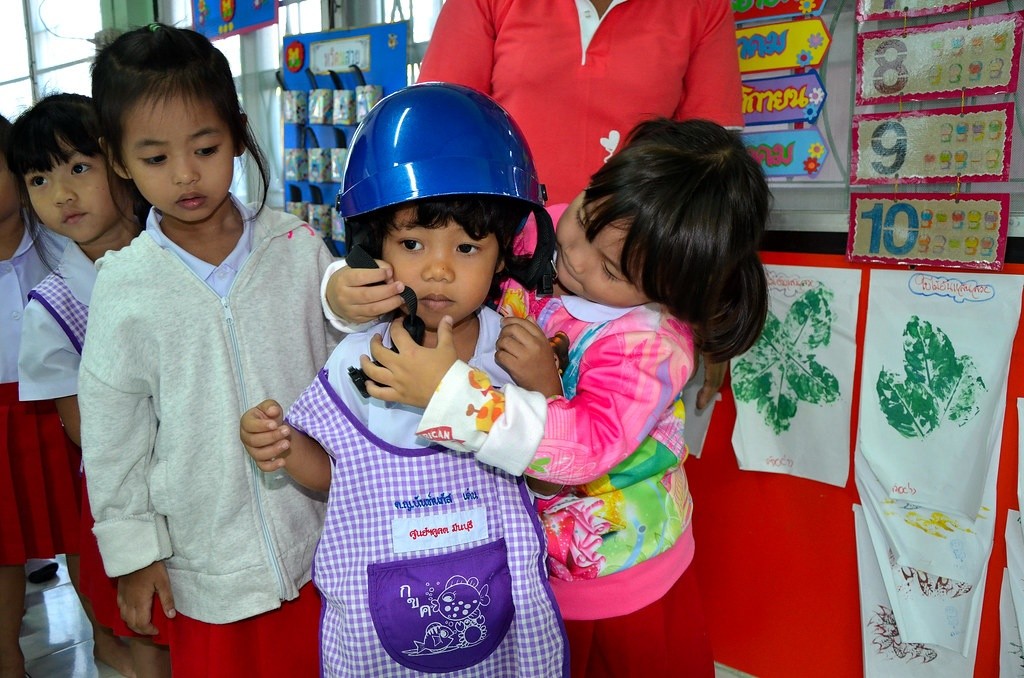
[420,1,773,626]
[6,90,173,675]
[73,19,352,677]
[234,80,581,675]
[0,109,151,678]
[310,115,775,675]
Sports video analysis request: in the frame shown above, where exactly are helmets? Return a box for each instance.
[334,81,547,222]
